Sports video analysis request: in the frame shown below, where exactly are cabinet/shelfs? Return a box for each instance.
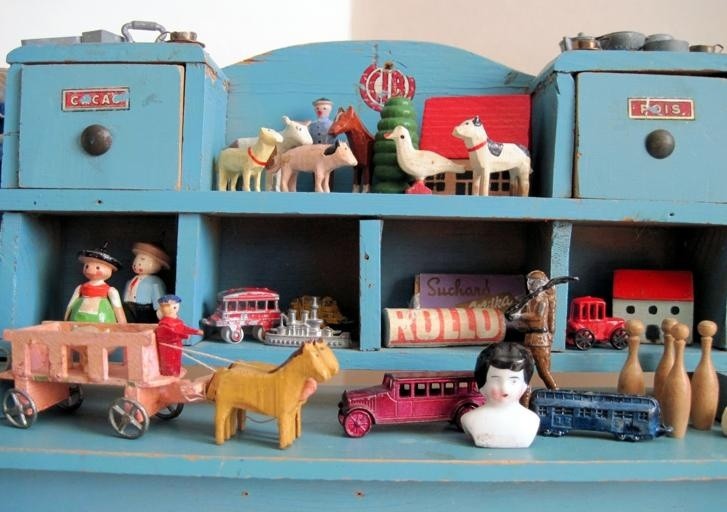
[0,37,727,512]
